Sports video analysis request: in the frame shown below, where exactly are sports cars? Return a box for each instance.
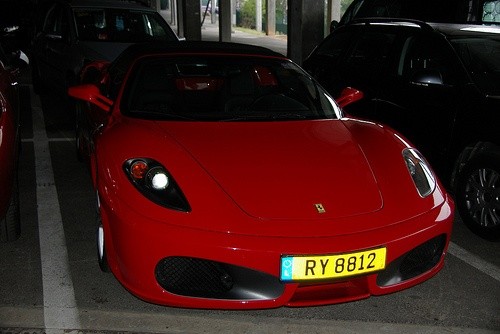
[73,41,453,312]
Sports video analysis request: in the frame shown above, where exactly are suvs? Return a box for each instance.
[30,2,188,97]
[299,17,499,245]
